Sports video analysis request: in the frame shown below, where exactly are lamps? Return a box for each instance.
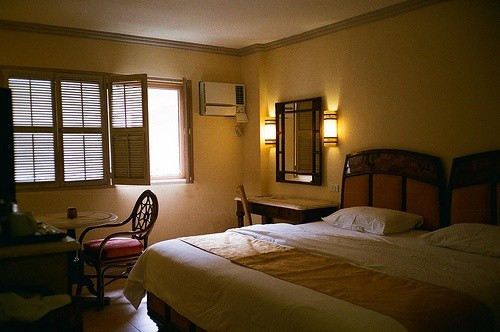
[264,117,276,145]
[323,111,338,147]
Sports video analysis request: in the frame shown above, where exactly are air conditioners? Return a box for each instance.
[199,81,248,122]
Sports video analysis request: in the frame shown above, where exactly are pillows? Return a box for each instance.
[418,222,500,257]
[320,205,424,236]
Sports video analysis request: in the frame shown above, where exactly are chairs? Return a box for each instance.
[74,189,159,310]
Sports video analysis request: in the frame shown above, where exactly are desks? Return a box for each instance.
[36,211,118,284]
[234,195,339,227]
[0,236,81,332]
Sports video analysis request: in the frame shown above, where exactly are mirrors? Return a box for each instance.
[275,96,322,187]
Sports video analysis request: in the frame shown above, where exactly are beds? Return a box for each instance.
[123,149,500,332]
